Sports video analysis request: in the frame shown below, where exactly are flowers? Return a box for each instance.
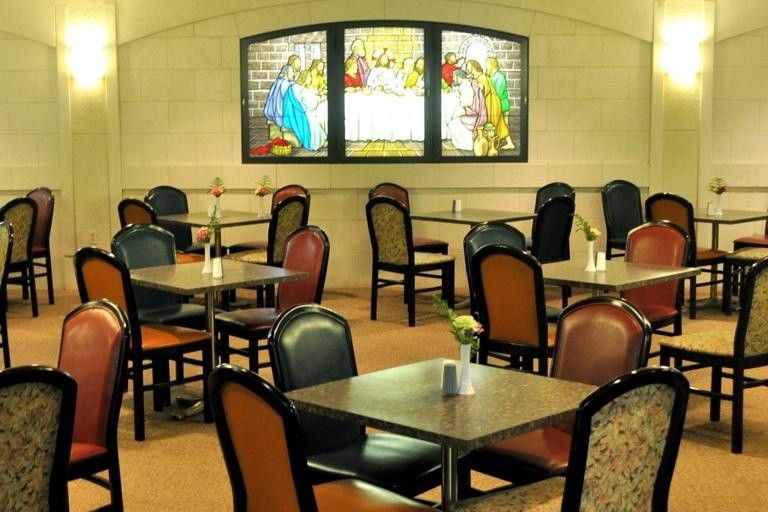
[429,294,484,343]
[572,211,600,242]
[197,204,220,244]
[207,176,228,196]
[254,176,274,195]
[707,177,725,194]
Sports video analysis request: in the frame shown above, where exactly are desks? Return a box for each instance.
[158,210,273,309]
[543,261,695,298]
[283,358,598,511]
[130,257,310,421]
[409,208,537,309]
[694,209,763,308]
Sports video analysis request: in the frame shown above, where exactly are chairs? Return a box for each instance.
[730,247,767,306]
[216,225,328,373]
[450,366,689,511]
[0,222,12,369]
[470,244,559,380]
[659,258,767,454]
[365,196,452,326]
[531,194,574,264]
[0,197,40,318]
[601,180,644,260]
[464,221,564,320]
[111,224,228,411]
[268,305,470,496]
[369,182,449,256]
[538,182,574,210]
[24,187,56,305]
[117,199,156,227]
[228,185,310,252]
[733,218,768,247]
[644,192,730,318]
[210,365,431,512]
[58,299,128,512]
[224,194,308,307]
[621,220,688,368]
[458,295,650,500]
[73,246,210,440]
[145,185,227,260]
[0,367,76,512]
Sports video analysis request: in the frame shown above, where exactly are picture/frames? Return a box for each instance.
[240,19,334,165]
[336,20,433,163]
[433,22,529,163]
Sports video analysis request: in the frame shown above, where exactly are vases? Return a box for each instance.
[585,238,597,271]
[705,180,728,217]
[256,196,266,219]
[715,196,723,214]
[215,197,221,217]
[458,346,477,396]
[202,244,212,273]
